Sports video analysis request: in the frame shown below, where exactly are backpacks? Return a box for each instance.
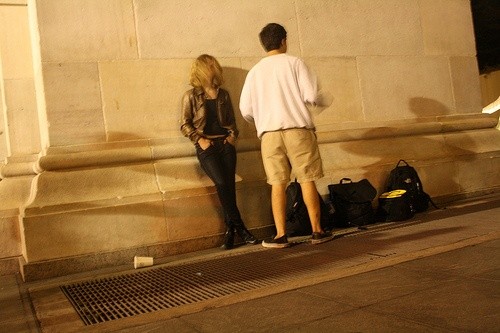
[282,177,329,235]
[386,158,440,214]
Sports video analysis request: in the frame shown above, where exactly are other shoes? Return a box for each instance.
[312,229,335,245]
[262,234,289,248]
[237,226,257,245]
[219,227,236,249]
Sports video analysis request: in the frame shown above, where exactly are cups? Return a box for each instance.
[133,256,153,269]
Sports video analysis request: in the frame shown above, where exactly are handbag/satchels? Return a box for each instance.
[378,189,417,221]
[328,177,377,227]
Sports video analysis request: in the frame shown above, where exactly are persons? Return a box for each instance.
[238,22,334,249]
[179,53,258,248]
